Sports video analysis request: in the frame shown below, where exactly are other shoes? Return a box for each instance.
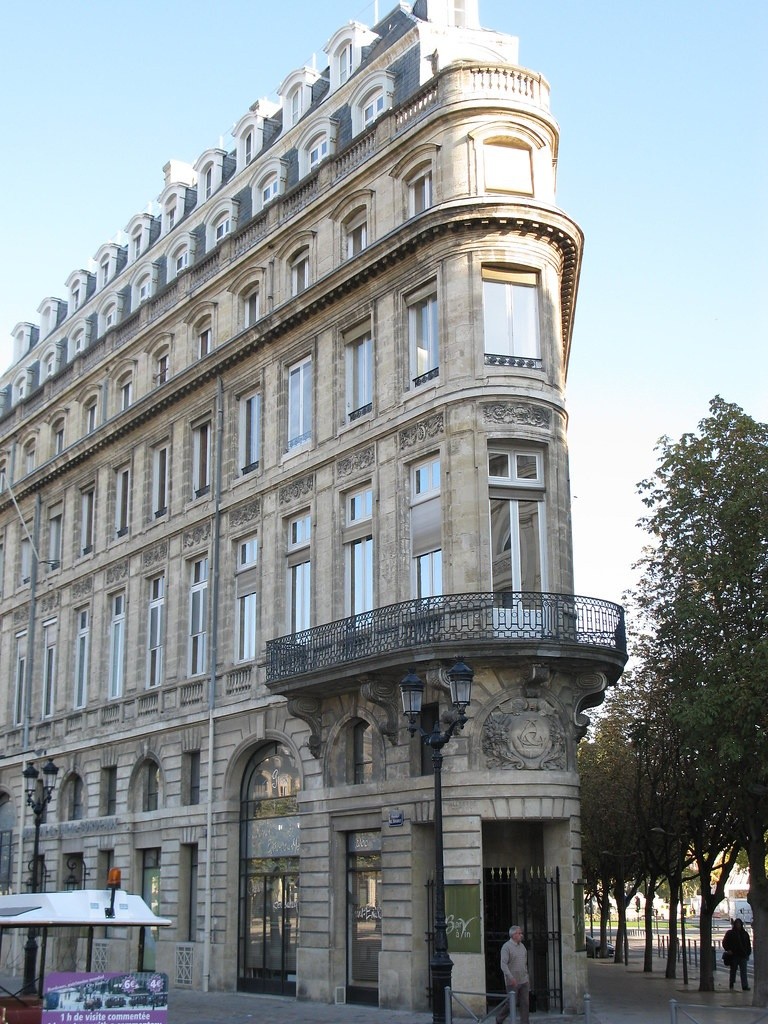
[730,983,734,989]
[743,987,750,991]
[496,1017,501,1024]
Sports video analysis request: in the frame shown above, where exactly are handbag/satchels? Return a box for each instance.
[722,952,730,966]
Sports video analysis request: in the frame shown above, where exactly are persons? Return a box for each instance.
[500,925,530,1024]
[722,919,751,991]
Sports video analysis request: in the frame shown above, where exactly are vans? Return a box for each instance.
[729,898,753,924]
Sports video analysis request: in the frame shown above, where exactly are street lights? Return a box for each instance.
[399,654,476,1024]
[20,757,59,995]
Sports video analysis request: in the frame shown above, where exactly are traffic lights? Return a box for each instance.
[635,900,640,910]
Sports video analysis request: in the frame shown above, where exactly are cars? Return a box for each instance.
[573,934,594,949]
[586,935,615,959]
